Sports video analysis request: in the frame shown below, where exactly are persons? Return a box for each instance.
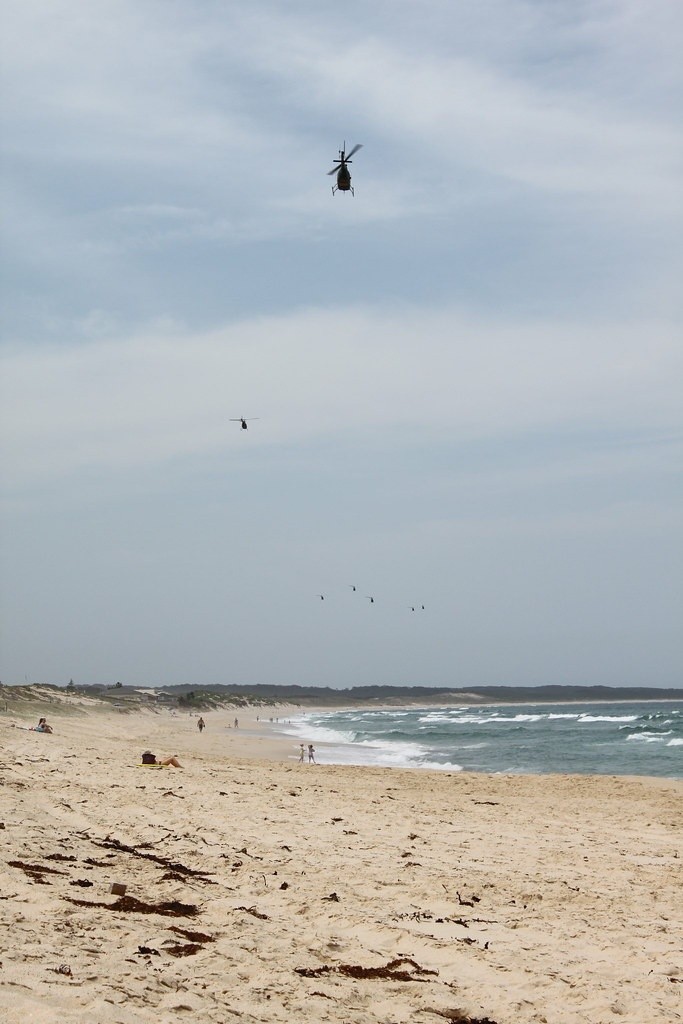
[142,750,184,769]
[308,745,316,763]
[197,717,205,733]
[234,718,239,728]
[298,744,306,763]
[36,717,53,734]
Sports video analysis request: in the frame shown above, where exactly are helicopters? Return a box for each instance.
[229,415,260,431]
[325,137,363,196]
